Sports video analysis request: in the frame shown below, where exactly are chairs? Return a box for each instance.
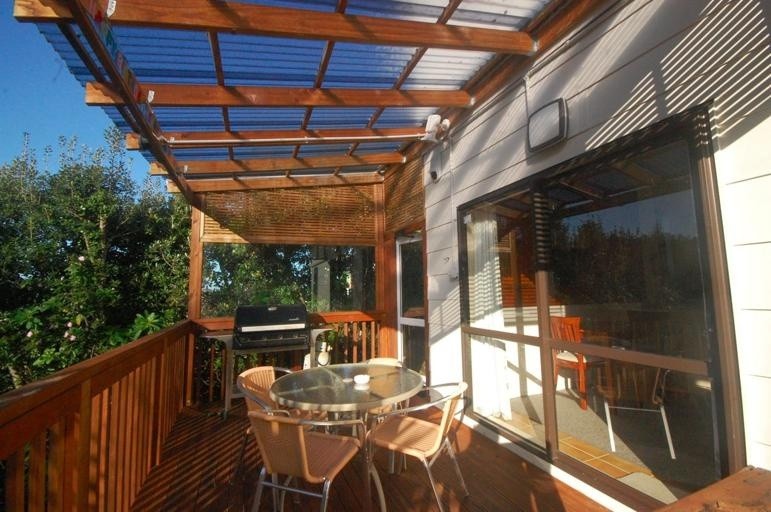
[233,355,468,512]
[542,310,657,417]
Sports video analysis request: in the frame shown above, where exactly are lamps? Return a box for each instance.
[527,97,569,154]
